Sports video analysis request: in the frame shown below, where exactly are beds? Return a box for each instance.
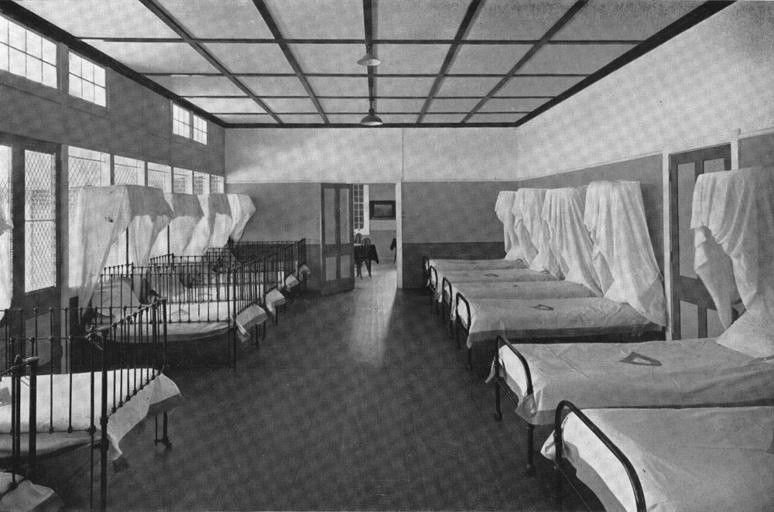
[438,277,605,336]
[79,238,311,369]
[1,357,64,512]
[427,266,565,310]
[0,297,184,511]
[454,292,667,369]
[424,255,529,304]
[551,401,773,512]
[492,334,774,475]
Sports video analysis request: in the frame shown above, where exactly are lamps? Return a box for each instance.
[360,96,384,128]
[356,43,382,66]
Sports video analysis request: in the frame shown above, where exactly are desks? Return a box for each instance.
[353,243,379,278]
[390,238,397,264]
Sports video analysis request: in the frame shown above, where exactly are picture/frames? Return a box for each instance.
[370,200,395,218]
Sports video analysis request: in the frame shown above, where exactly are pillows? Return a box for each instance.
[605,278,630,305]
[566,267,582,285]
[530,255,545,272]
[505,248,517,261]
[716,308,774,363]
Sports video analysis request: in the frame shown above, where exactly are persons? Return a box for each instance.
[353,233,364,280]
[363,237,378,279]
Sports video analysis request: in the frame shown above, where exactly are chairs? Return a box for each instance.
[355,238,372,280]
[355,234,363,243]
